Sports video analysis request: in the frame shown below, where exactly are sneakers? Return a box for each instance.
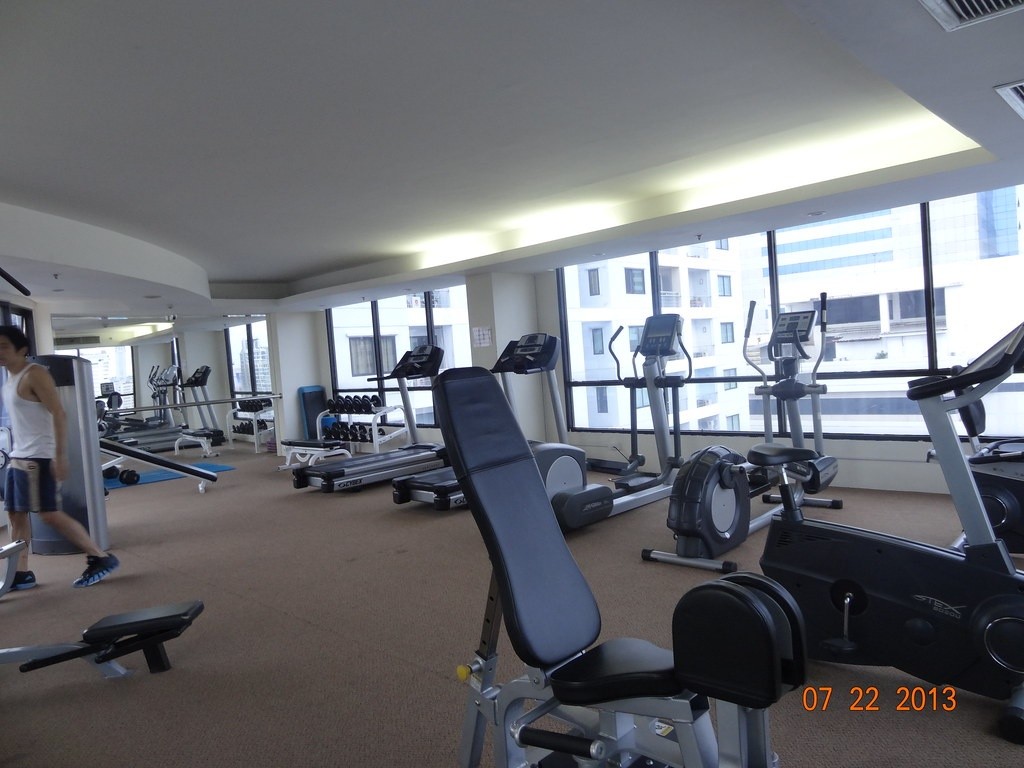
[5,570,37,590]
[72,553,120,589]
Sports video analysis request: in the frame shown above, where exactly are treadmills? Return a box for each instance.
[96,364,226,454]
[292,332,568,510]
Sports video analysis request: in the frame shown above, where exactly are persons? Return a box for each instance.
[0,323,120,593]
